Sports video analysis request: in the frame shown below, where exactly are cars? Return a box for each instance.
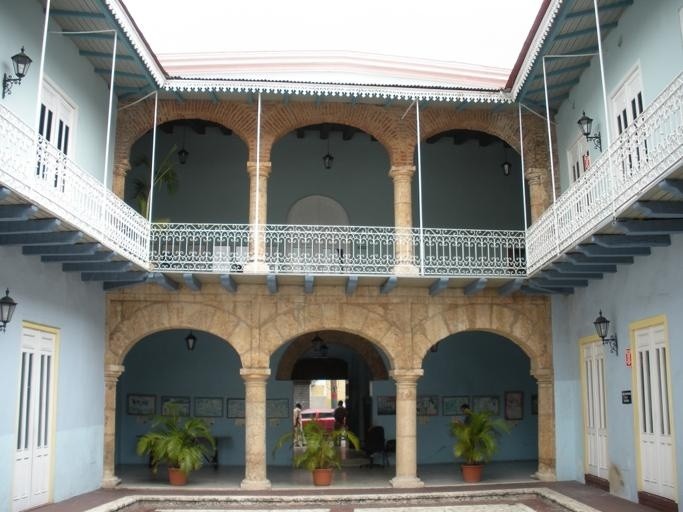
[299,406,346,431]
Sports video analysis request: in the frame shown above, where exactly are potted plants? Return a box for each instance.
[135,403,217,485]
[271,423,360,486]
[446,407,514,482]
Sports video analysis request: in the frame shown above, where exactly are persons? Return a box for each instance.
[460,402,471,424]
[293,402,307,447]
[332,400,347,447]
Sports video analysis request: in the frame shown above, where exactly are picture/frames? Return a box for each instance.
[417,391,538,420]
[127,393,289,419]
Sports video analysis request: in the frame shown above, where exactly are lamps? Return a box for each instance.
[593,310,619,356]
[185,330,196,352]
[322,131,334,169]
[178,121,188,164]
[311,333,323,352]
[431,341,441,352]
[577,110,601,152]
[502,150,512,176]
[0,287,17,333]
[2,45,32,99]
